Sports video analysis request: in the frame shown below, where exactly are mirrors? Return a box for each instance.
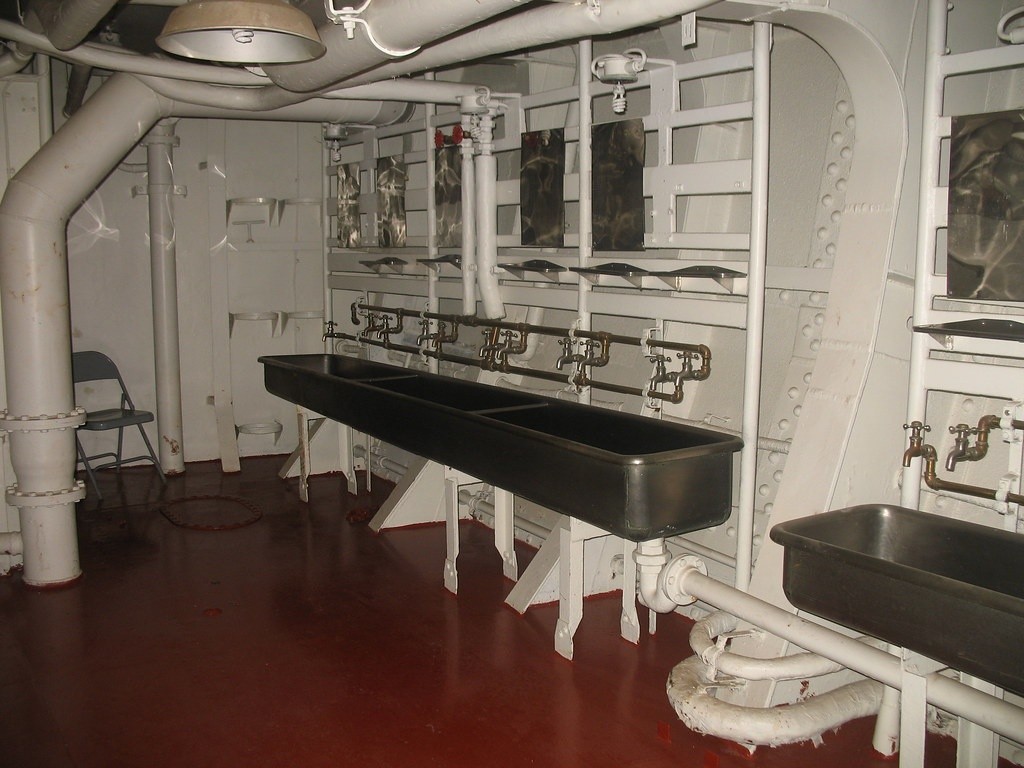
[948,109,1024,302]
[591,119,646,251]
[336,161,361,248]
[376,153,406,248]
[520,127,566,247]
[436,145,463,247]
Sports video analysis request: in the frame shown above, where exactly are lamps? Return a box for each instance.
[591,48,647,112]
[154,1,327,64]
[325,124,347,161]
[455,86,491,138]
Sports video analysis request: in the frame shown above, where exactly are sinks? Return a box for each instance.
[770,504,1024,699]
[361,376,542,464]
[257,353,415,417]
[478,404,745,543]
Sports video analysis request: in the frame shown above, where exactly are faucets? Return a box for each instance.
[364,313,378,337]
[322,321,338,342]
[479,328,497,357]
[417,319,433,346]
[377,314,393,339]
[497,330,518,359]
[676,350,698,387]
[432,322,447,347]
[578,340,600,371]
[902,420,931,468]
[945,423,977,472]
[556,338,577,370]
[650,355,672,390]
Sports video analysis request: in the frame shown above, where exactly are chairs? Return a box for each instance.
[71,351,168,498]
